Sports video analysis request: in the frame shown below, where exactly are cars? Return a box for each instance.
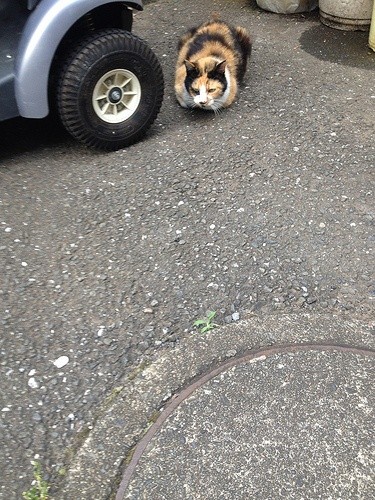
[0,0,165,148]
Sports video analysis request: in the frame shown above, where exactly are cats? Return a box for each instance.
[174,19,252,118]
